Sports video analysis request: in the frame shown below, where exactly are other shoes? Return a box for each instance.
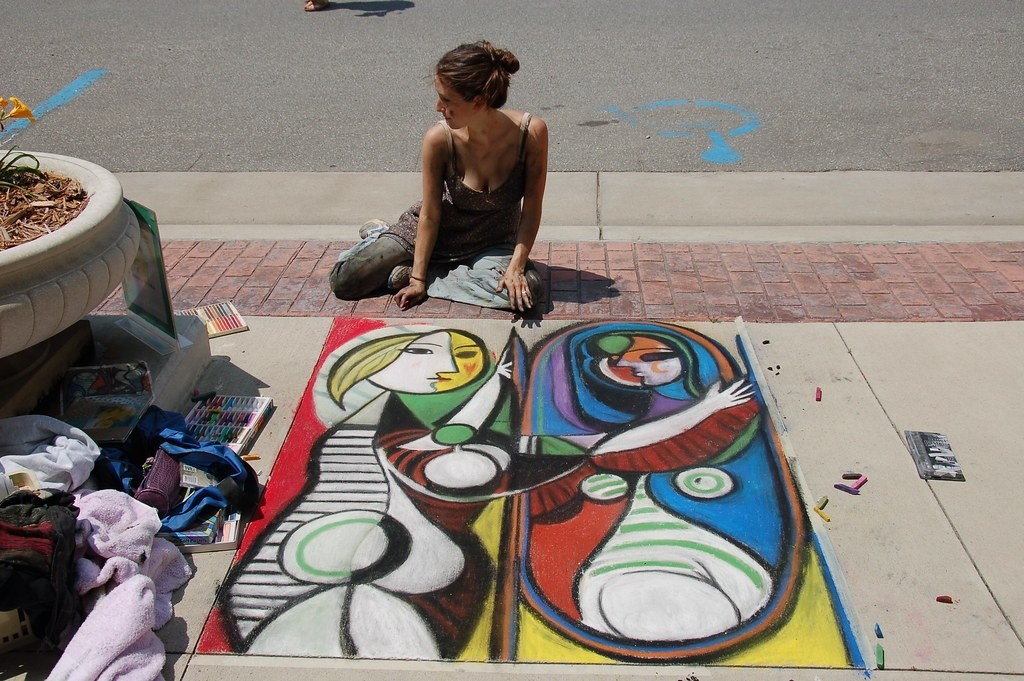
[387,259,413,295]
[304,0,329,11]
[358,218,390,239]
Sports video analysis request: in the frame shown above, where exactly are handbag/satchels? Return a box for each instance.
[90,405,260,533]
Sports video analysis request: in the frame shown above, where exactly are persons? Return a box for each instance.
[304,0,329,11]
[329,40,549,311]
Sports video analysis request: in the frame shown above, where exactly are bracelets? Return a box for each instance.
[410,276,425,282]
